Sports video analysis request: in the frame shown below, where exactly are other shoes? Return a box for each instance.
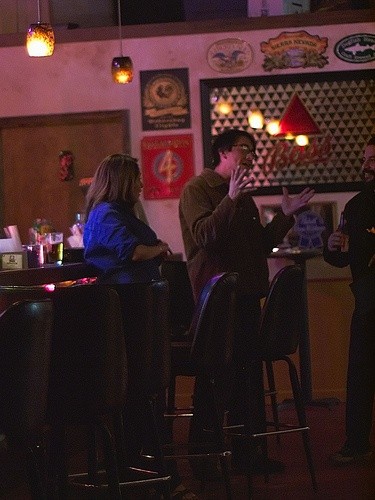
[232,458,283,473]
[193,466,218,480]
[327,450,368,464]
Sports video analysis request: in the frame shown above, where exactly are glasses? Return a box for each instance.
[229,145,255,161]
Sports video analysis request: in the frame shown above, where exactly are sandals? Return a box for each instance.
[128,484,198,500]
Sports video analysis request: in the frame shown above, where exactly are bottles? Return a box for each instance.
[336,211,350,253]
[74,212,87,238]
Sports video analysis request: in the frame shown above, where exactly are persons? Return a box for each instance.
[322,137,375,465]
[178,130,317,483]
[82,154,182,487]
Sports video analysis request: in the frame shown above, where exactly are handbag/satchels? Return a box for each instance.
[161,250,195,309]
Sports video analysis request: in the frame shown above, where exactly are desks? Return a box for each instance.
[0,247,191,413]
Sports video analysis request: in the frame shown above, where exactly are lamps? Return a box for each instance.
[27,0,55,58]
[111,0,135,84]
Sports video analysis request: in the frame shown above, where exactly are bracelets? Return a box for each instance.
[158,244,163,253]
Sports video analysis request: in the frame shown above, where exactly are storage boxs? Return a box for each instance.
[259,200,339,250]
[247,0,311,17]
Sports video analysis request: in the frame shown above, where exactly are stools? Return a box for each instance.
[124,278,173,500]
[0,300,53,500]
[62,287,122,500]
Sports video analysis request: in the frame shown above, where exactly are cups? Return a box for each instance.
[28,245,45,267]
[46,232,65,266]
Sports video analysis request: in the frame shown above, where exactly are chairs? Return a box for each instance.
[139,266,321,500]
